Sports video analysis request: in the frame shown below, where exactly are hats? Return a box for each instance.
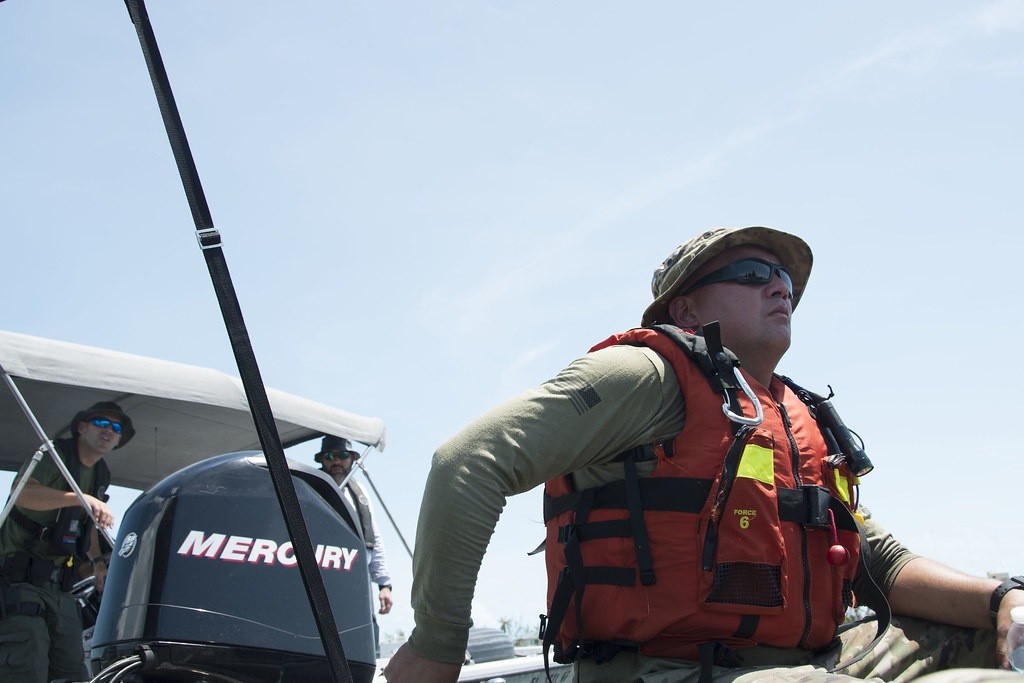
[70,401,135,450]
[641,227,813,327]
[315,436,360,462]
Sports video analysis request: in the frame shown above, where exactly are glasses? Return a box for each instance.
[84,417,123,434]
[320,451,353,460]
[677,257,794,298]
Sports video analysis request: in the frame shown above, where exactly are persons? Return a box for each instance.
[386,229,1023,680]
[384,225,1024,683]
[315,435,394,659]
[1,402,137,683]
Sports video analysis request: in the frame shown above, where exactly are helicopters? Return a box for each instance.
[0,0,570,682]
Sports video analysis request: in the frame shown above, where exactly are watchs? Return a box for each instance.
[379,584,392,592]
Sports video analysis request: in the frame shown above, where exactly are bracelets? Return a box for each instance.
[91,557,107,566]
[987,576,1023,640]
